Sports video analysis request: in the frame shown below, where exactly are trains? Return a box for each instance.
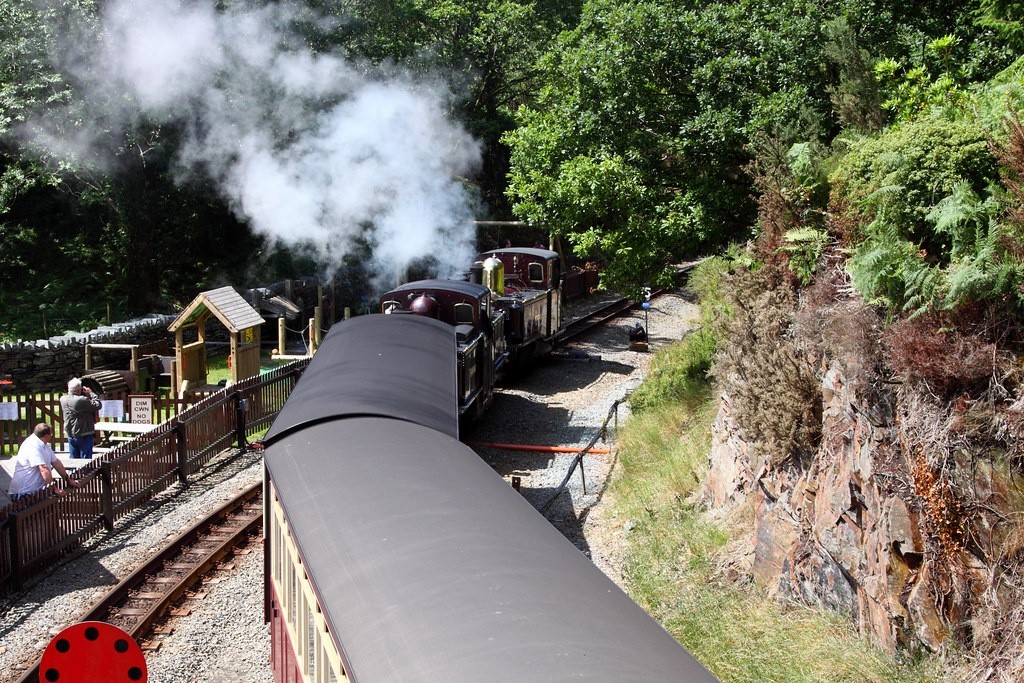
[262,246,719,683]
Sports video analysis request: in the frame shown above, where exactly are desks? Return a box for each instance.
[58,458,92,469]
[94,422,157,447]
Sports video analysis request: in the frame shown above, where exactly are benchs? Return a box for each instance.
[94,447,112,454]
[102,436,136,441]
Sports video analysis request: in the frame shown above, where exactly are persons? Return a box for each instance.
[60,377,102,459]
[8,423,80,556]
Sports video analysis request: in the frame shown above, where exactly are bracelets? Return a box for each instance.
[65,473,69,481]
